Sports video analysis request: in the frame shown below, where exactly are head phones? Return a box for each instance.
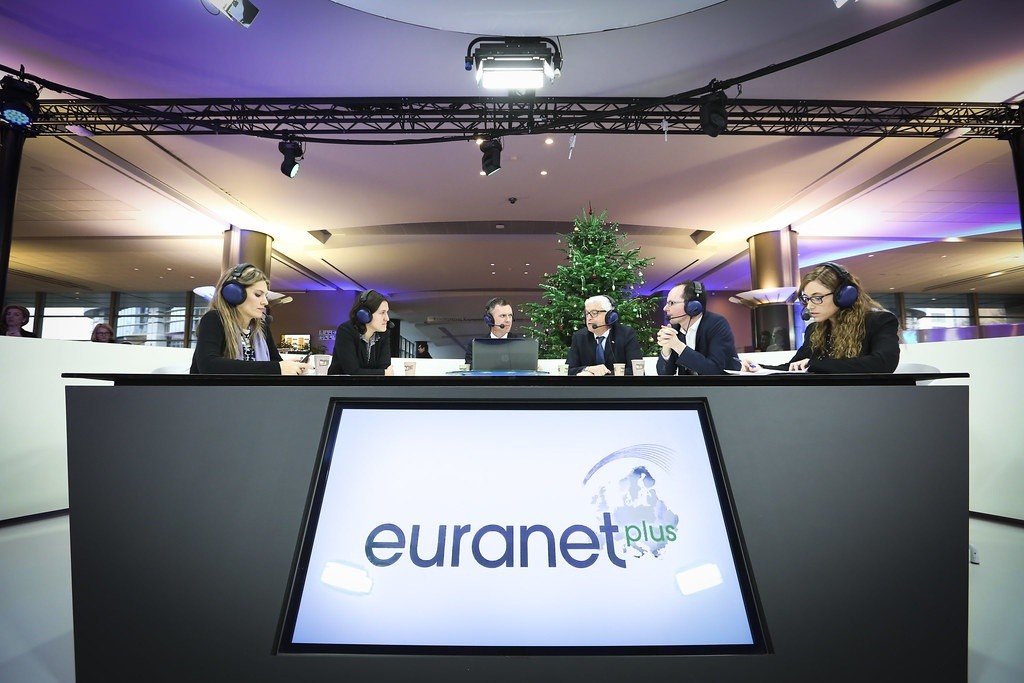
[221,264,256,306]
[605,295,620,326]
[484,298,515,327]
[817,262,860,309]
[355,289,375,323]
[684,281,703,317]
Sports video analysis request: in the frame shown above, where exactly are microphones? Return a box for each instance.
[800,306,810,321]
[592,321,616,329]
[388,321,395,329]
[262,313,273,322]
[490,324,505,328]
[665,312,694,321]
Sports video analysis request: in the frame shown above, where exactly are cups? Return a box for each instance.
[631,360,645,376]
[314,355,330,375]
[404,362,416,375]
[558,364,568,375]
[613,364,625,376]
[459,364,469,371]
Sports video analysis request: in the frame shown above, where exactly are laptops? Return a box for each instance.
[472,338,540,371]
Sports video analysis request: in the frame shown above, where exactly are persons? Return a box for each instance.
[766,326,788,352]
[190,263,314,376]
[465,297,519,371]
[417,343,432,359]
[742,262,900,373]
[0,304,39,338]
[565,295,646,376]
[755,330,770,352]
[656,281,742,374]
[91,323,116,343]
[327,289,396,376]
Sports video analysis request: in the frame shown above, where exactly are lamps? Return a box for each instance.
[480,140,503,176]
[278,142,303,177]
[0,75,40,126]
[465,36,563,90]
[699,91,727,138]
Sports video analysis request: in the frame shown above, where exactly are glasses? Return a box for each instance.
[799,292,834,305]
[666,301,687,307]
[584,310,607,318]
[96,332,111,336]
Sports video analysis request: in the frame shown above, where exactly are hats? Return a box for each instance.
[3,305,30,326]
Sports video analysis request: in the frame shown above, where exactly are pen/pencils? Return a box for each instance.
[732,357,755,367]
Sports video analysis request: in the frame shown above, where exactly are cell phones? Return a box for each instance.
[301,352,313,363]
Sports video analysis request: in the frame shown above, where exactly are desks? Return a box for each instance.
[62,372,970,683]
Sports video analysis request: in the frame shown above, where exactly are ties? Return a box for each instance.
[595,337,605,365]
